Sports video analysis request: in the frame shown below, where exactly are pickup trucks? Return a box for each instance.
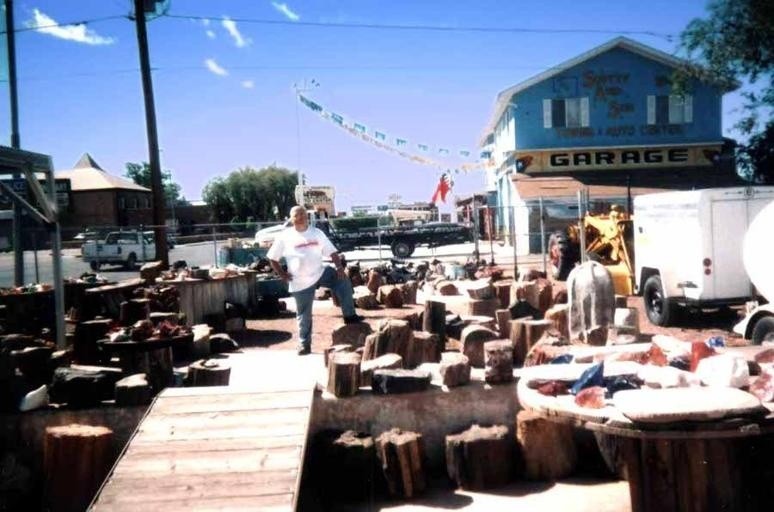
[81,230,156,271]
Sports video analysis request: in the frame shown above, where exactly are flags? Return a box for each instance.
[296,93,496,173]
[437,170,451,203]
[431,183,442,206]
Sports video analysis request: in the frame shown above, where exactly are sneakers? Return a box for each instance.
[296,346,311,356]
[343,313,366,325]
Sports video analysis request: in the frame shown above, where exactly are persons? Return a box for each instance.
[265,205,362,355]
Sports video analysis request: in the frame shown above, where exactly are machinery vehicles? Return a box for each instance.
[547,198,634,296]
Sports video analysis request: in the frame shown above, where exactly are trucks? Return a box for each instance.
[255,209,466,259]
